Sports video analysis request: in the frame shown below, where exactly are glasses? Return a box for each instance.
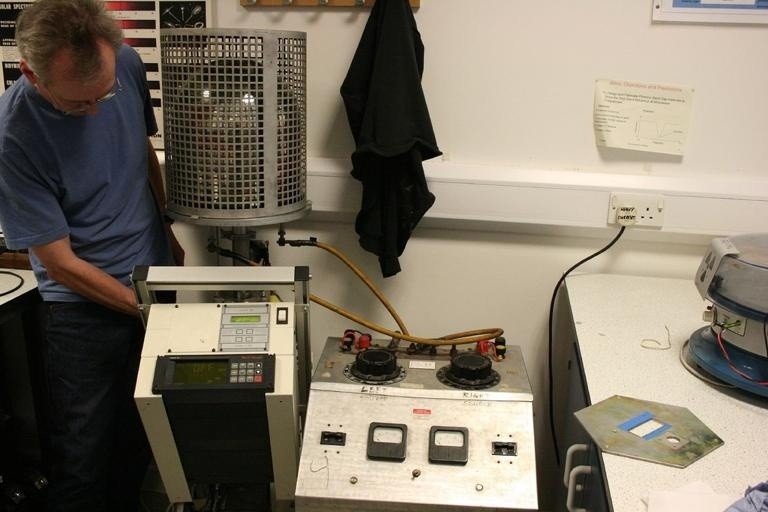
[36,75,122,113]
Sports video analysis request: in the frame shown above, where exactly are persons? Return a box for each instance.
[1,1,189,510]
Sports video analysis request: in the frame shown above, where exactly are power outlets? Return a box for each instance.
[607,190,667,228]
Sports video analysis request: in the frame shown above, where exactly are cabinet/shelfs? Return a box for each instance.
[561,271,768,511]
